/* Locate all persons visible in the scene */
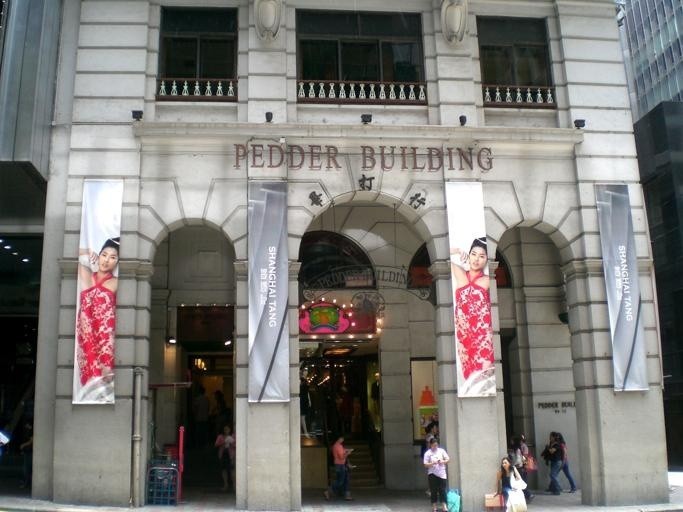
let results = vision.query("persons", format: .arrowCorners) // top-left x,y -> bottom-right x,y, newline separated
518,433 -> 530,456
299,374 -> 312,435
422,422 -> 439,501
507,434 -> 534,504
423,437 -> 449,512
333,393 -> 343,416
449,235 -> 496,398
74,236 -> 119,405
495,457 -> 528,512
544,431 -> 577,494
322,434 -> 357,501
214,423 -> 237,494
545,431 -> 564,496
16,417 -> 33,491
370,371 -> 380,434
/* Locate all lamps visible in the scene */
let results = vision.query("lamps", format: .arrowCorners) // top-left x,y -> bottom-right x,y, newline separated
265,112 -> 274,124
132,110 -> 143,121
362,113 -> 372,125
250,0 -> 282,40
459,115 -> 466,126
436,0 -> 471,43
574,119 -> 585,130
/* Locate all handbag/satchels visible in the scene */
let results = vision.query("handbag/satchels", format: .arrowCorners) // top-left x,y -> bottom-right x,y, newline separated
447,489 -> 460,512
510,471 -> 528,490
